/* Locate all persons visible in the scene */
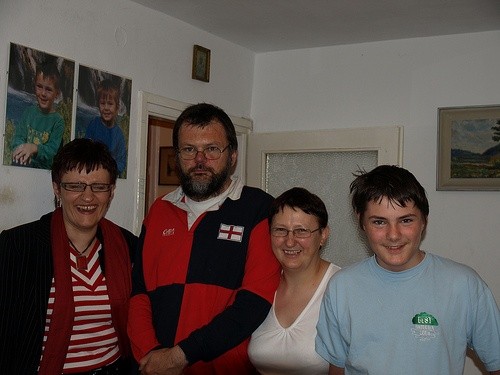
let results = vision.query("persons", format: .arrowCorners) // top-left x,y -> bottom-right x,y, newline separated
315,165 -> 500,375
247,187 -> 343,375
12,62 -> 64,170
127,103 -> 282,375
85,78 -> 126,174
0,138 -> 139,374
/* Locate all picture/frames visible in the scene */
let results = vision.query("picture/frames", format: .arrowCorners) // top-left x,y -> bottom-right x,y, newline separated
436,105 -> 500,192
158,146 -> 180,186
191,44 -> 211,82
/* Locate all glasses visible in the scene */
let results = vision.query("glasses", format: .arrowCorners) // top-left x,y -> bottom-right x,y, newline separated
60,183 -> 112,192
270,228 -> 320,238
177,144 -> 229,160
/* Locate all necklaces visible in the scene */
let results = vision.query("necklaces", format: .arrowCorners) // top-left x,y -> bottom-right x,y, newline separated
69,234 -> 96,270
